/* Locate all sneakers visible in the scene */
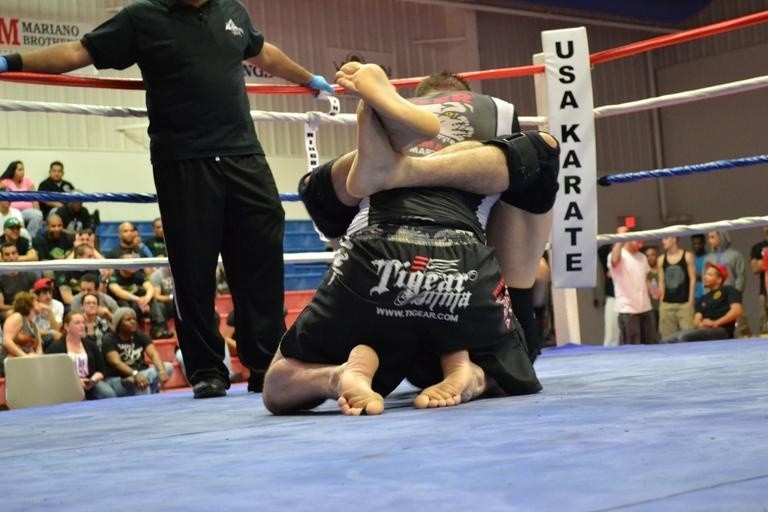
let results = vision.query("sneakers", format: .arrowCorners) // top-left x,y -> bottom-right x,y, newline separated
151,327 -> 174,339
120,376 -> 135,389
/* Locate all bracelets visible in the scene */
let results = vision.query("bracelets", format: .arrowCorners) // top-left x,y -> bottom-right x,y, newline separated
3,52 -> 22,73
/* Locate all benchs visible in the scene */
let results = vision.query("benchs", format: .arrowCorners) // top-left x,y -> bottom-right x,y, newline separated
1,217 -> 335,406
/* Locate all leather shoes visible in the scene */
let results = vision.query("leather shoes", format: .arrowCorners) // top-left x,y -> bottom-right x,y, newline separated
193,378 -> 227,398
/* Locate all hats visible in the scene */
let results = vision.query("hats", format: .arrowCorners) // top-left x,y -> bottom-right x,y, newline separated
32,278 -> 55,292
4,217 -> 22,228
705,260 -> 728,280
112,307 -> 136,330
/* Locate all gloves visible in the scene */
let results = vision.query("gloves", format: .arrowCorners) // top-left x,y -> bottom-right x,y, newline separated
307,74 -> 335,97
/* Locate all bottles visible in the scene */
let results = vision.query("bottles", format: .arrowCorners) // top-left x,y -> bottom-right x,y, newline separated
650,278 -> 658,300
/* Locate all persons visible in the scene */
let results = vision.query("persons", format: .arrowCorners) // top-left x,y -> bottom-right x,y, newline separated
1,161 -> 74,237
297,63 -> 562,386
605,220 -> 768,343
1,186 -> 287,400
2,0 -> 335,400
